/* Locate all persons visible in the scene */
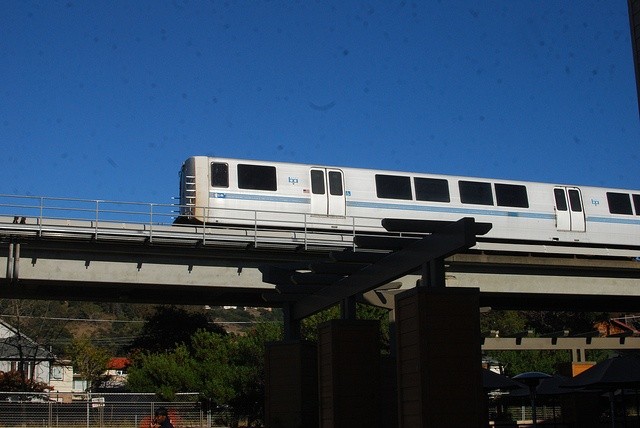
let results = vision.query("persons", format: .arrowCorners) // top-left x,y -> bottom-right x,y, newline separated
155,408 -> 174,428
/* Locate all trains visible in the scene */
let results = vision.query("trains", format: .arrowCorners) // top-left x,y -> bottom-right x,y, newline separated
170,155 -> 640,264
